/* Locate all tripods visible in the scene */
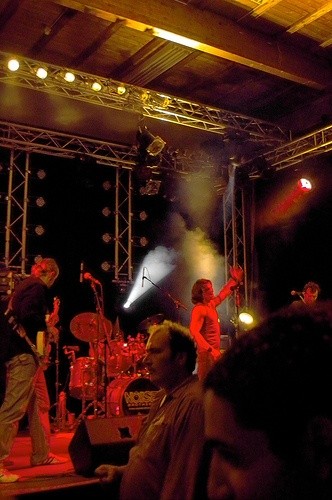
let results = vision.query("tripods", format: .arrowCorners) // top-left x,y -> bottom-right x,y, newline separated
47,282 -> 114,433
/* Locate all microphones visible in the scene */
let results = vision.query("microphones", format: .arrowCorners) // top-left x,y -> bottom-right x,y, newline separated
84,272 -> 101,286
291,291 -> 307,296
142,268 -> 144,287
79,262 -> 83,282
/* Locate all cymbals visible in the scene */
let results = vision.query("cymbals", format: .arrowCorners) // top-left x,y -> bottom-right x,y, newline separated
138,314 -> 163,330
69,312 -> 113,343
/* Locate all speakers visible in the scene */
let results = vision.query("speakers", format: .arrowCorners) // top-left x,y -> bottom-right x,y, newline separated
69,415 -> 147,479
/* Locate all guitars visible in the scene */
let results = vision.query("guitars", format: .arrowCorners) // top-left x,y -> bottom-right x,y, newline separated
36,297 -> 60,370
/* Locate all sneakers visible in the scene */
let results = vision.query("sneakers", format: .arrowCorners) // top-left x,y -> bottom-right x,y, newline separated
0,468 -> 19,483
31,452 -> 68,465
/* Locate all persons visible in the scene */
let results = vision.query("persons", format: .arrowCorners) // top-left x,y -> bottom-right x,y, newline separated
204,307 -> 332,500
95,321 -> 205,500
0,258 -> 60,483
189,265 -> 243,381
289,282 -> 320,307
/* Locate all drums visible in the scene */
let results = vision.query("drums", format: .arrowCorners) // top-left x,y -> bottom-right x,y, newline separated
88,338 -> 148,377
103,375 -> 160,416
69,357 -> 105,399
